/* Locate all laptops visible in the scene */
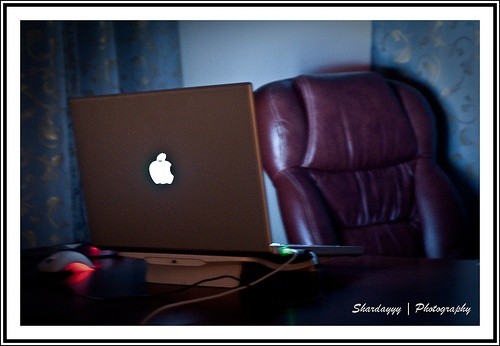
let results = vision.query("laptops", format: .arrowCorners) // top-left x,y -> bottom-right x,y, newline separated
65,78 -> 364,271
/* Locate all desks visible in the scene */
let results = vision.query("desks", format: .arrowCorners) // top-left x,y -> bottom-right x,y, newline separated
20,243 -> 479,325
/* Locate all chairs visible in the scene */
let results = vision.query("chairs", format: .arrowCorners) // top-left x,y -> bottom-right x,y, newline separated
252,72 -> 461,260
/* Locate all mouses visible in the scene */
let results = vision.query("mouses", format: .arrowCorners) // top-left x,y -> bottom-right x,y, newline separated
34,247 -> 98,278
